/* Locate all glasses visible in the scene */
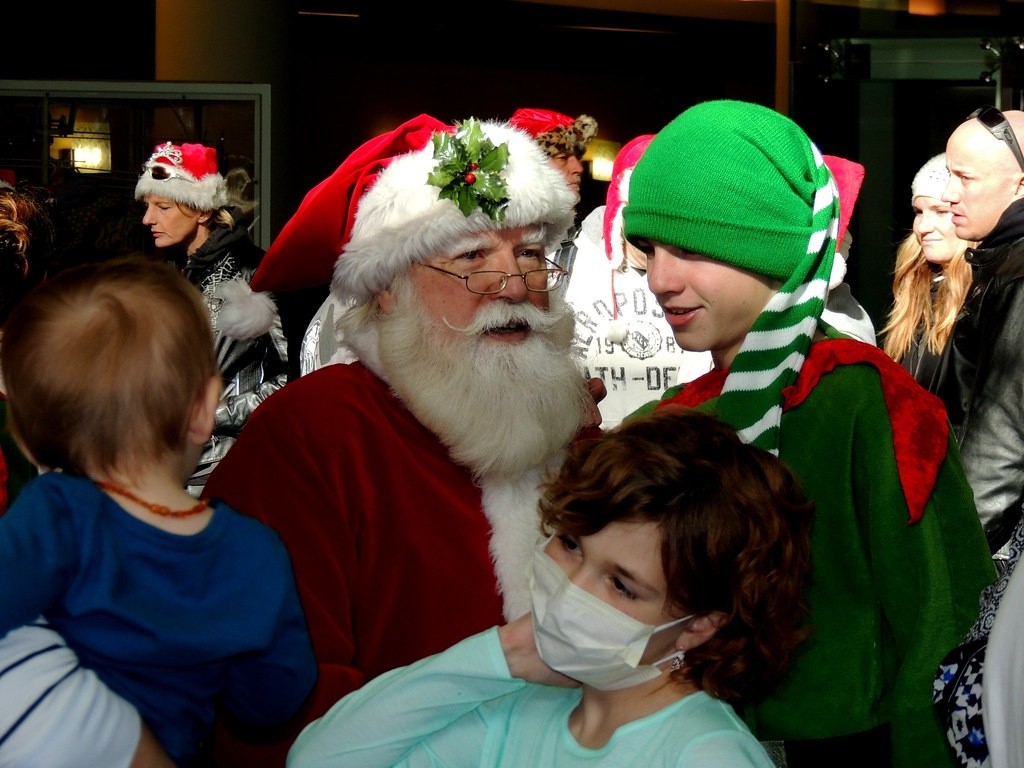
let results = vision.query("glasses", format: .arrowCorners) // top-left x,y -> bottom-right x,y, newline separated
139,163 -> 195,182
969,105 -> 1023,173
415,257 -> 571,294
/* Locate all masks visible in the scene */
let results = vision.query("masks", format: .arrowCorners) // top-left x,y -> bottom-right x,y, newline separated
523,530 -> 693,691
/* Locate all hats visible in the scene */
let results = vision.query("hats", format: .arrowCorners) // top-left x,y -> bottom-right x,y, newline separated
910,151 -> 950,201
822,154 -> 866,290
511,107 -> 596,155
217,111 -> 578,342
605,133 -> 656,268
134,143 -> 228,209
622,97 -> 843,462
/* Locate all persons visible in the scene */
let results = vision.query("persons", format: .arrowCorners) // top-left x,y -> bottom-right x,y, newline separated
287,411 -> 819,768
134,142 -> 302,498
203,113 -> 592,768
936,105 -> 1024,560
884,152 -> 982,390
0,255 -> 319,768
507,106 -> 599,201
585,99 -> 1000,768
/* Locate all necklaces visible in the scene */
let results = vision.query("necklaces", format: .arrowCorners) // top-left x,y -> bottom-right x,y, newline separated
97,481 -> 209,518
